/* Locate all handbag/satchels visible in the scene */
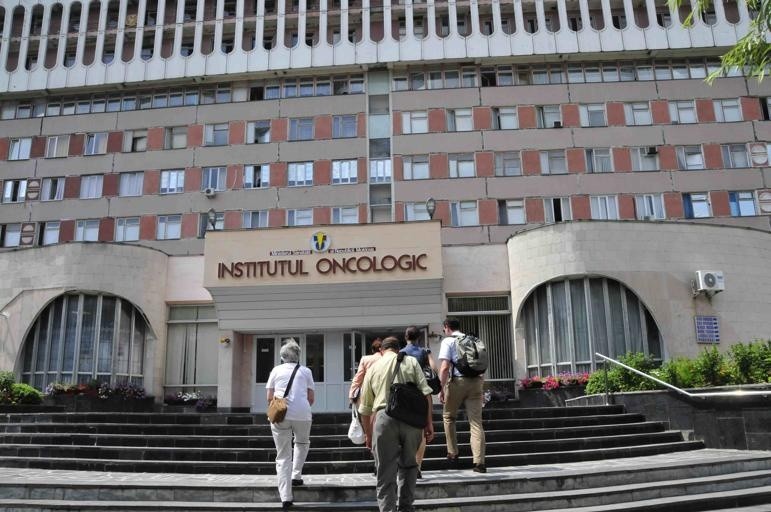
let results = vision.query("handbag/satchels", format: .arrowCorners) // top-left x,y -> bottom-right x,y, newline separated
348,403 -> 366,445
385,382 -> 428,429
422,366 -> 442,395
348,387 -> 360,409
267,397 -> 287,424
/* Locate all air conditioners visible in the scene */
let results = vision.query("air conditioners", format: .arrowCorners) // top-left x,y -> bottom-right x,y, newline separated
693,269 -> 725,293
648,146 -> 658,153
204,187 -> 216,197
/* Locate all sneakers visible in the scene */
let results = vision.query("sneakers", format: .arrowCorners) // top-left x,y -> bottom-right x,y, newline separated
283,501 -> 293,505
417,469 -> 422,478
447,454 -> 458,460
473,465 -> 487,473
292,479 -> 304,486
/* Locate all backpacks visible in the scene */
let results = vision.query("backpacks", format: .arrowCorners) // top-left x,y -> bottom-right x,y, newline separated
446,333 -> 488,375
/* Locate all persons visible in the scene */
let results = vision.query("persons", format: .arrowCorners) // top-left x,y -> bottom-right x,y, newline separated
358,336 -> 434,512
399,325 -> 437,478
265,337 -> 315,507
439,316 -> 488,473
348,339 -> 384,475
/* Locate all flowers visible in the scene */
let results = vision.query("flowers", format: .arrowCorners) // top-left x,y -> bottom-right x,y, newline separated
518,370 -> 589,391
46,378 -> 214,411
